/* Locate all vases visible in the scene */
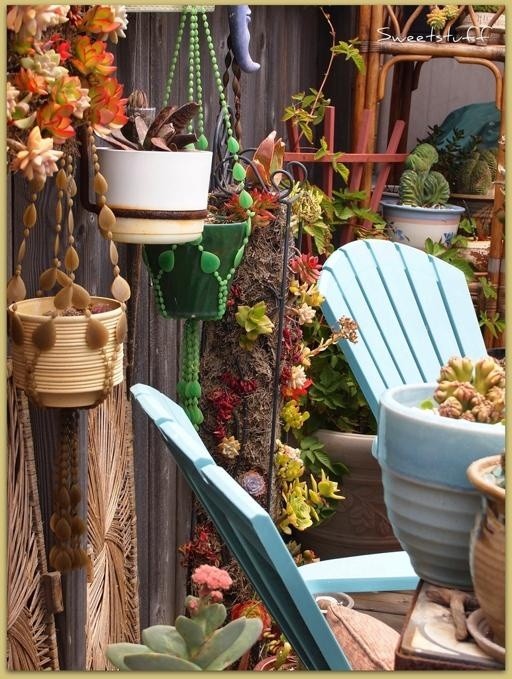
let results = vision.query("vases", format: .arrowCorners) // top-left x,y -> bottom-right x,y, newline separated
7,294 -> 126,410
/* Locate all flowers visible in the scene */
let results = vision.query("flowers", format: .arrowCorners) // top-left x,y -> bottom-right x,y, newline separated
6,4 -> 128,181
